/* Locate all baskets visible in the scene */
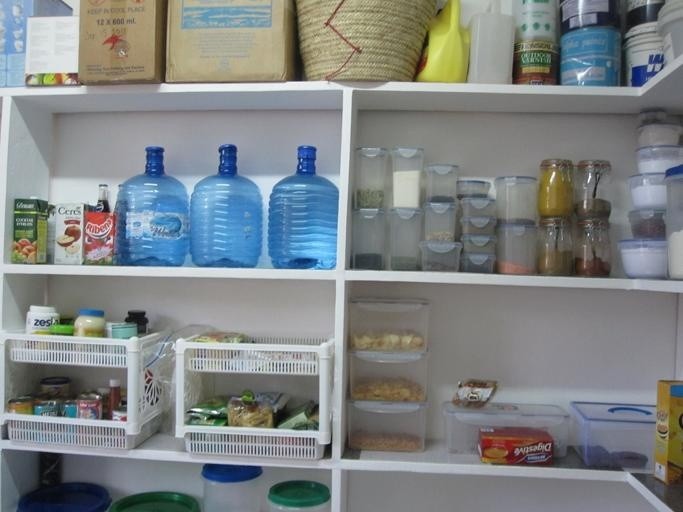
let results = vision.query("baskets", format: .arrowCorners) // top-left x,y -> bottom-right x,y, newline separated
297,0 -> 438,82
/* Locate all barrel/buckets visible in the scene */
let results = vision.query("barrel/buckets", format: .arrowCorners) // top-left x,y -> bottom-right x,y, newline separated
623,24 -> 662,86
468,0 -> 516,83
202,463 -> 266,512
417,2 -> 468,82
657,0 -> 683,65
16,483 -> 108,512
266,480 -> 331,511
109,491 -> 200,512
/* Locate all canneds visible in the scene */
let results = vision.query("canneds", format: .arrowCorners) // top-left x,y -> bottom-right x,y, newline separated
112,405 -> 127,421
538,158 -> 613,277
8,376 -> 103,419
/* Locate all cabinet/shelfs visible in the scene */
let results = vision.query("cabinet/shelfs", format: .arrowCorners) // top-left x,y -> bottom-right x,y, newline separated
0,54 -> 683,512
77,0 -> 297,86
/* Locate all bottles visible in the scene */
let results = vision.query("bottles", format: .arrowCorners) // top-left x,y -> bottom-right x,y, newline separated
107,376 -> 122,420
95,183 -> 111,214
572,157 -> 613,219
571,220 -> 614,278
535,157 -> 576,218
26,304 -> 149,341
534,214 -> 575,277
